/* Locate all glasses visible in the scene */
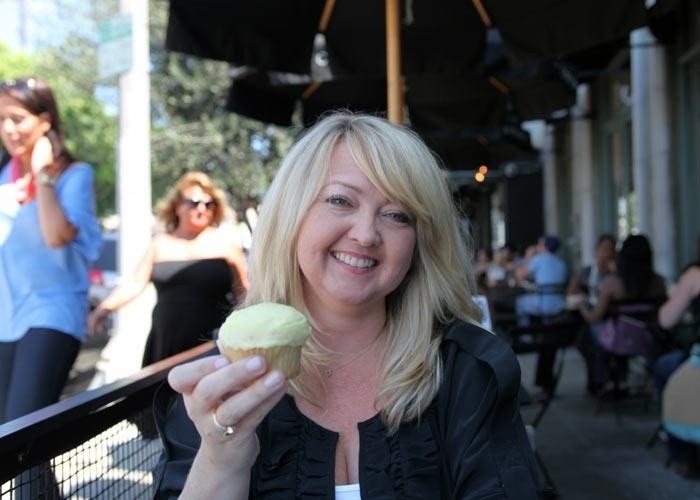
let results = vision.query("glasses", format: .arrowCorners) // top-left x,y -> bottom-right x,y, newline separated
182,199 -> 214,211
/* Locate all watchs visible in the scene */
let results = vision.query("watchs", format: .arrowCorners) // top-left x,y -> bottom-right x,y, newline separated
33,174 -> 54,186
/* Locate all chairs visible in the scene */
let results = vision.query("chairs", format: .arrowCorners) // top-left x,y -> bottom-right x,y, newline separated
484,283 -> 699,477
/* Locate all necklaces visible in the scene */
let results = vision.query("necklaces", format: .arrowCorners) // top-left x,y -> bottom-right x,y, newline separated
319,342 -> 372,376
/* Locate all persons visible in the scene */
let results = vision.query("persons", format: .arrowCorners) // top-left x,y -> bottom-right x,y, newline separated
153,109 -> 560,500
474,235 -> 699,477
87,171 -> 249,440
0,78 -> 103,500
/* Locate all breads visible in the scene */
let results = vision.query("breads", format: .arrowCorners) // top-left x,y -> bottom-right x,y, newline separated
216,300 -> 311,380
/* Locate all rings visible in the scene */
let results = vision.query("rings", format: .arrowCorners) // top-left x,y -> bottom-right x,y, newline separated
213,409 -> 237,437
